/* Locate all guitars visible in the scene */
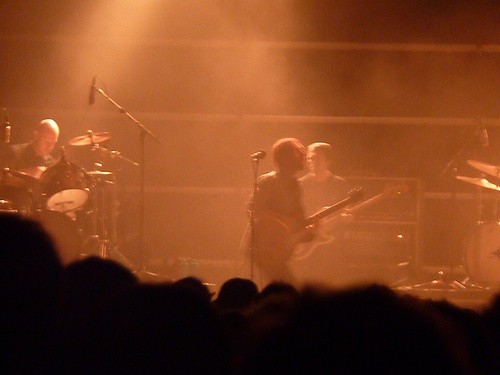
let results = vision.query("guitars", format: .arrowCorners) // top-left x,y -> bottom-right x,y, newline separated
253,183 -> 407,261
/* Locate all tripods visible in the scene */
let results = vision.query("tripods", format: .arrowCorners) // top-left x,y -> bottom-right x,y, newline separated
80,176 -> 130,268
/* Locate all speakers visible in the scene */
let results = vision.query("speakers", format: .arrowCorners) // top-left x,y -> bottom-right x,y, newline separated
332,219 -> 418,290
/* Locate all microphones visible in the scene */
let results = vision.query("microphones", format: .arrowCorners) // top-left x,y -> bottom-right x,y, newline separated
480,123 -> 489,146
60,146 -> 65,158
89,77 -> 97,105
4,113 -> 11,143
249,150 -> 266,160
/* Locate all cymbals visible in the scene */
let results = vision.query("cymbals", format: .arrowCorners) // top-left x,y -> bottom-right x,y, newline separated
68,132 -> 112,146
467,159 -> 500,178
78,169 -> 123,178
455,175 -> 500,191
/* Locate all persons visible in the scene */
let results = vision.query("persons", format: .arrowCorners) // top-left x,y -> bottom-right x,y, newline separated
240,138 -> 322,282
298,142 -> 353,284
0,211 -> 500,375
5,118 -> 60,170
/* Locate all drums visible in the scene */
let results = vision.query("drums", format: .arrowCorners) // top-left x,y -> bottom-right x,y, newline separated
46,188 -> 96,239
464,219 -> 500,287
13,209 -> 82,265
